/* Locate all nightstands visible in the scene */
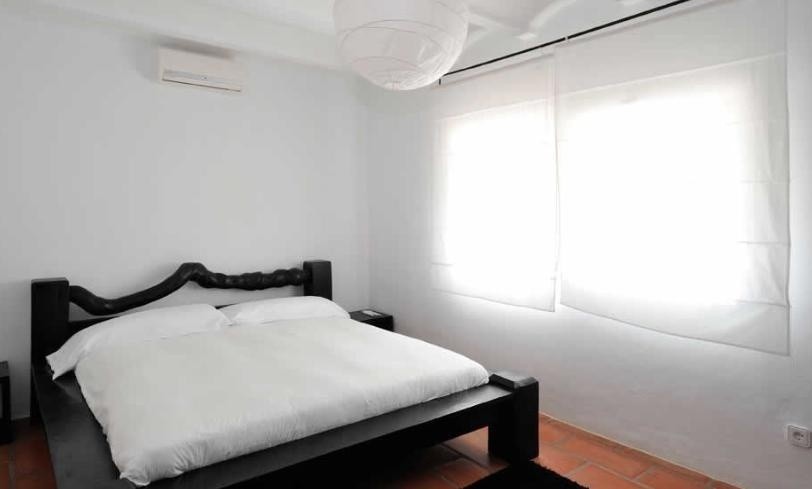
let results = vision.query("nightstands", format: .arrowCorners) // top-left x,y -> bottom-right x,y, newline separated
0,360 -> 14,445
348,309 -> 393,331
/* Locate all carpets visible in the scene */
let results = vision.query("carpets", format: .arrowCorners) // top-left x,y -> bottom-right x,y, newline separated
462,459 -> 589,489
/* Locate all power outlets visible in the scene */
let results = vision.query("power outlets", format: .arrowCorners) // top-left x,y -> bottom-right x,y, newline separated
785,424 -> 812,448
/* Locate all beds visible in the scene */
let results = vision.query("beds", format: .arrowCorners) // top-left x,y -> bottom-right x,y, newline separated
29,259 -> 539,489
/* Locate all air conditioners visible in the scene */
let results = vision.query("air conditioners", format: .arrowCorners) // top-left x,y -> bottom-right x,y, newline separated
156,48 -> 243,95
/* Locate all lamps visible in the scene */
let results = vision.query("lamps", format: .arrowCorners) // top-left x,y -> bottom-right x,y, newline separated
330,0 -> 468,90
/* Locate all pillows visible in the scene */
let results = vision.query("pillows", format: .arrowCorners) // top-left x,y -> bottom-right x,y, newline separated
45,303 -> 232,381
217,296 -> 351,327
